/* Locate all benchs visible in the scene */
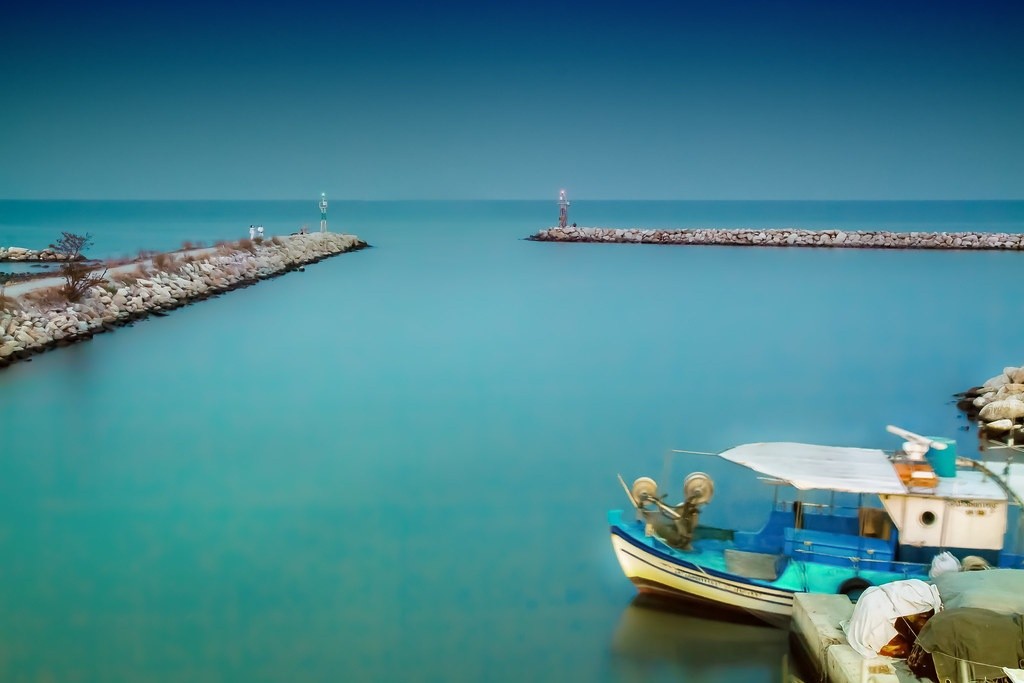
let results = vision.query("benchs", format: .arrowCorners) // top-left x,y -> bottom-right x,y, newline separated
782,527 -> 891,569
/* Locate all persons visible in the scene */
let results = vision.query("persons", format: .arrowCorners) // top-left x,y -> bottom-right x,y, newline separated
257,224 -> 264,237
248,224 -> 256,240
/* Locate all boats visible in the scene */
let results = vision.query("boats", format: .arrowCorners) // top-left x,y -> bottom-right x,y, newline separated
607,424 -> 1023,629
